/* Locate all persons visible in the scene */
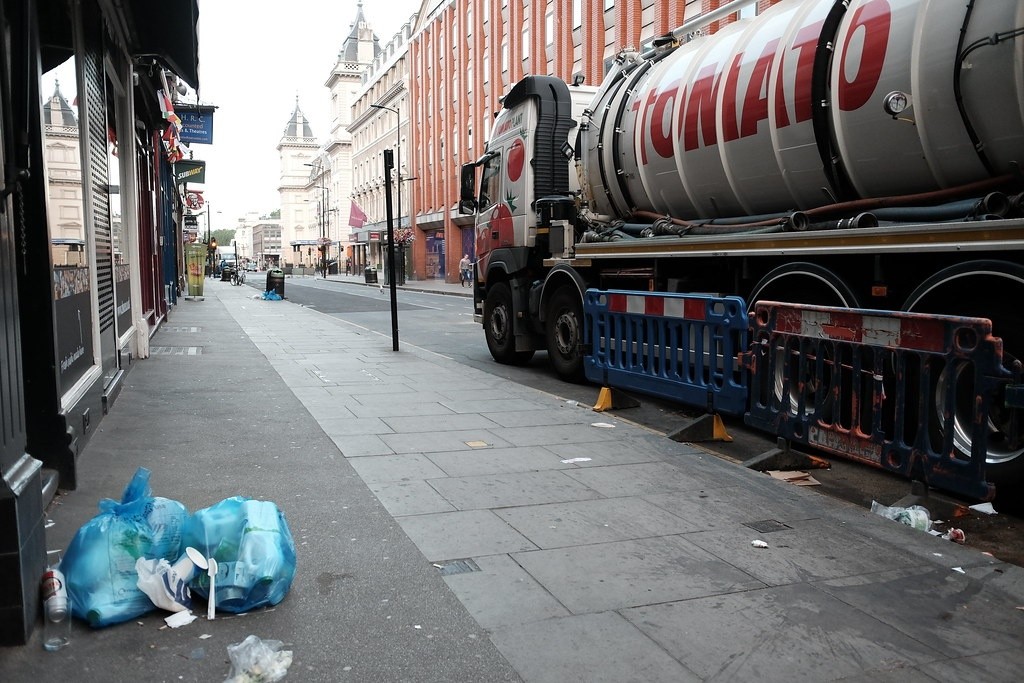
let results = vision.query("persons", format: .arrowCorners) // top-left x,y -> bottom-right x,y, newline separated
215,259 -> 226,277
459,254 -> 473,288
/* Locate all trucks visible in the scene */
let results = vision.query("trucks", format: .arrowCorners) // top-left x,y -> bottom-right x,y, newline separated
218,252 -> 240,271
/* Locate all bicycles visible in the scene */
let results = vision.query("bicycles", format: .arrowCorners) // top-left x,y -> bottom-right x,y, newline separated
231,270 -> 246,287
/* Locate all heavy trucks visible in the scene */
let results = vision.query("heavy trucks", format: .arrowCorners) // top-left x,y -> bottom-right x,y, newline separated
459,0 -> 1024,512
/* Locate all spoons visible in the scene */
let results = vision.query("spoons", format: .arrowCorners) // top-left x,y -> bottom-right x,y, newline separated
207,558 -> 218,621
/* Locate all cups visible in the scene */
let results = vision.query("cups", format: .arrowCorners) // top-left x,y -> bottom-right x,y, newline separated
171,547 -> 207,584
43,596 -> 73,651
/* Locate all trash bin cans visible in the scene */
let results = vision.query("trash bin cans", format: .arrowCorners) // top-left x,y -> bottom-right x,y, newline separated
219,266 -> 231,281
364,267 -> 378,283
266,268 -> 284,300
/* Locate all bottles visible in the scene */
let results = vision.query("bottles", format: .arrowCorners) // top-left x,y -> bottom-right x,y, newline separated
42,570 -> 68,621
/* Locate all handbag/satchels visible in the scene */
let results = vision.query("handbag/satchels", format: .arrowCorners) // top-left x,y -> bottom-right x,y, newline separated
179,494 -> 300,613
59,466 -> 188,629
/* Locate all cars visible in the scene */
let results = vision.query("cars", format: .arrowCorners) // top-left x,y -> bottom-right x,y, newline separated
246,262 -> 257,272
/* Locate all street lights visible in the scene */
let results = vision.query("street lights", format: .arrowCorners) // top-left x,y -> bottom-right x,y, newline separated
370,104 -> 402,229
301,163 -> 335,279
